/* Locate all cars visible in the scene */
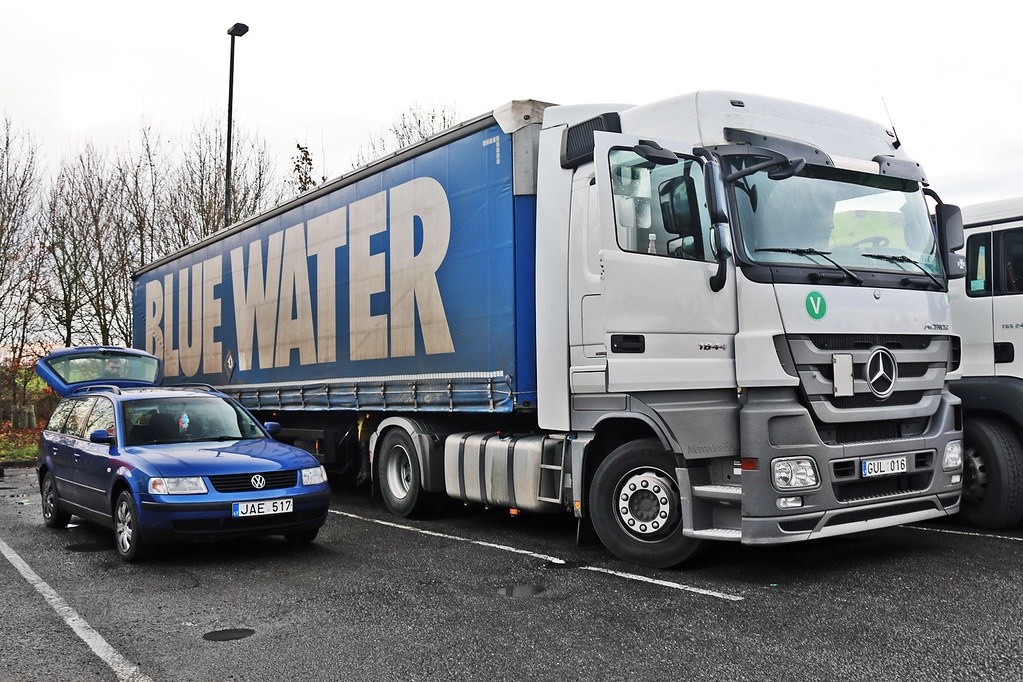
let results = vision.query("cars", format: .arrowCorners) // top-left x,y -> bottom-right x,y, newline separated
35,345 -> 331,563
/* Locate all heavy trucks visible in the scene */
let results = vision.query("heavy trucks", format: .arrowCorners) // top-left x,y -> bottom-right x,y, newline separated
129,90 -> 1023,567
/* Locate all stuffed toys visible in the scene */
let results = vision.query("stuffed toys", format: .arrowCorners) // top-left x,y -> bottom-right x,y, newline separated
179,414 -> 189,429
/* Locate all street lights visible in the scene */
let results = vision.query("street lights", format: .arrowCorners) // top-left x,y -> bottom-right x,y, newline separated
224,23 -> 249,228
